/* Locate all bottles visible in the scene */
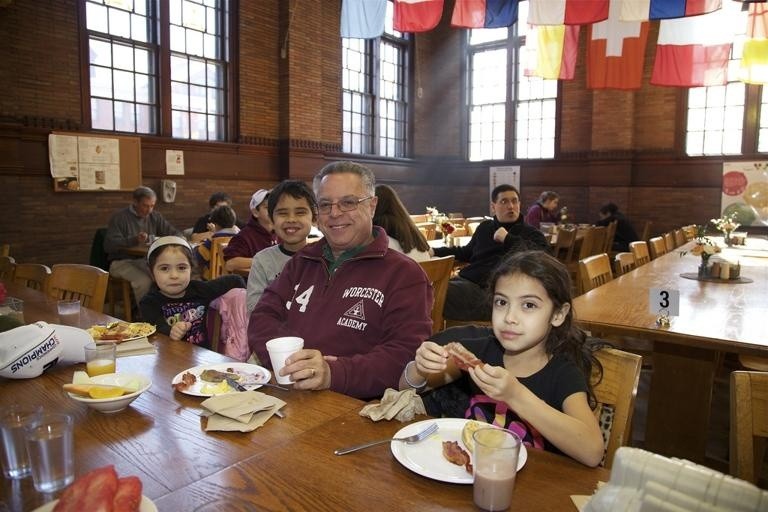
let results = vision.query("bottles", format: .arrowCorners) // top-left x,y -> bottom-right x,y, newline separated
710,262 -> 730,281
447,233 -> 461,247
732,235 -> 741,245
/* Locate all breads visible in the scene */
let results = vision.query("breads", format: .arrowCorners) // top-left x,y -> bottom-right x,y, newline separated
460,419 -> 501,457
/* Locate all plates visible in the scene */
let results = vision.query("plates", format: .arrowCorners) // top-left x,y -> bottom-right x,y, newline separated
389,417 -> 528,487
31,493 -> 159,512
94,321 -> 157,344
170,362 -> 272,399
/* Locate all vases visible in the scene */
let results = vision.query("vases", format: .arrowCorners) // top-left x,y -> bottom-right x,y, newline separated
698,262 -> 710,279
723,234 -> 734,247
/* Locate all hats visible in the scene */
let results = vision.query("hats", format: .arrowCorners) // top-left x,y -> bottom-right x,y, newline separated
249,189 -> 274,210
0,321 -> 96,380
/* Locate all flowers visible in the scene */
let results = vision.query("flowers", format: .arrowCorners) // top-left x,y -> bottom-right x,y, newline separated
677,235 -> 722,261
708,210 -> 743,234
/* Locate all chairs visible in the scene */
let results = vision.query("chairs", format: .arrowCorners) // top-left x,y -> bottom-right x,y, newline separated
584,342 -> 643,470
569,222 -> 702,296
107,209 -> 624,359
725,367 -> 767,486
1,256 -> 110,314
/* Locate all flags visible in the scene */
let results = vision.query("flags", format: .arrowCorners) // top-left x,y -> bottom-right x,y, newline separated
335,1 -> 768,89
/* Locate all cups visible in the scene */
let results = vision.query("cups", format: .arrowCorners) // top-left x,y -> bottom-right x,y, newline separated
56,299 -> 81,328
263,335 -> 305,387
84,340 -> 117,377
469,427 -> 521,512
0,403 -> 76,494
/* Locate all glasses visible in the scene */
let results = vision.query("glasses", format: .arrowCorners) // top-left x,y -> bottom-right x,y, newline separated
316,195 -> 373,215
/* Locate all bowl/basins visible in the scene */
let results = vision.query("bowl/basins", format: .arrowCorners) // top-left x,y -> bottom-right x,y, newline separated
66,371 -> 154,414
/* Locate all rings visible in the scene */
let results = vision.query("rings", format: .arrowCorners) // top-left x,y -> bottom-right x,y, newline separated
310,369 -> 316,378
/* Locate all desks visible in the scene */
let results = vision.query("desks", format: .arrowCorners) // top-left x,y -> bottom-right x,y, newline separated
1,277 -> 611,512
569,233 -> 768,469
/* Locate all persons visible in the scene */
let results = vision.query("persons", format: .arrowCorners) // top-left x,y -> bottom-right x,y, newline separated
248,182 -> 319,316
590,202 -> 638,259
223,188 -> 280,271
426,184 -> 553,323
106,186 -> 186,322
247,162 -> 434,399
139,237 -> 247,352
396,249 -> 605,468
190,192 -> 247,282
524,190 -> 570,231
372,183 -> 430,262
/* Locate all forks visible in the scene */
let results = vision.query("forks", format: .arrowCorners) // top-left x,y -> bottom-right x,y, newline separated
331,423 -> 440,457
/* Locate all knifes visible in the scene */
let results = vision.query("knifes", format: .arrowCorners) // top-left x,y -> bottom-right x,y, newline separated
224,375 -> 290,421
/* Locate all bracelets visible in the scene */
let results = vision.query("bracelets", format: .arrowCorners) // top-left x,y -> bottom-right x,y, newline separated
402,360 -> 429,389
